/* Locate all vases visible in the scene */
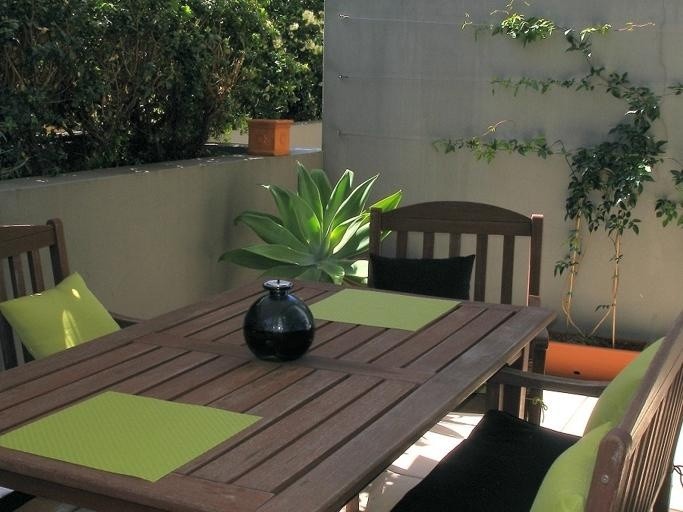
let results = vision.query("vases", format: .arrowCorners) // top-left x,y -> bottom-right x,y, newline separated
242,279 -> 314,360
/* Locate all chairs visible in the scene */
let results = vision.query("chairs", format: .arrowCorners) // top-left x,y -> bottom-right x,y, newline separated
0,217 -> 141,369
393,308 -> 682,511
344,203 -> 548,510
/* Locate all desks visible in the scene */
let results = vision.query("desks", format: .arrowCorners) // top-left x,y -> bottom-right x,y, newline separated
0,283 -> 555,511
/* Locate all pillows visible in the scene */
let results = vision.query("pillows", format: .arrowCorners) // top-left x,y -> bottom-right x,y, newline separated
364,252 -> 472,300
0,273 -> 121,363
581,336 -> 665,438
528,421 -> 612,512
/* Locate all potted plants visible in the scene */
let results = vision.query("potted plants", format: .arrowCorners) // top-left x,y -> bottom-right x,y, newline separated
420,0 -> 682,380
231,46 -> 311,157
